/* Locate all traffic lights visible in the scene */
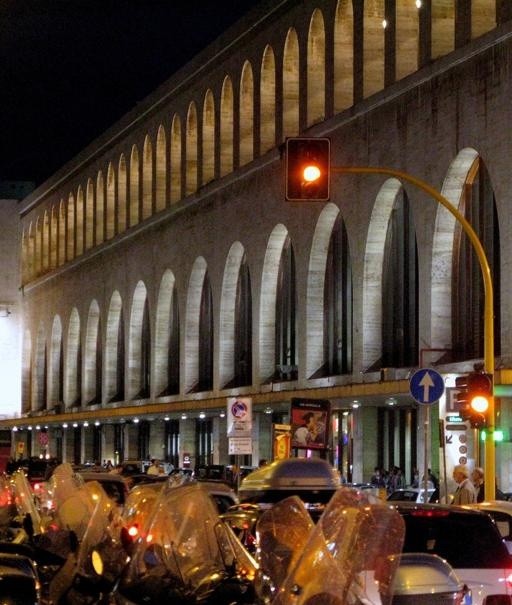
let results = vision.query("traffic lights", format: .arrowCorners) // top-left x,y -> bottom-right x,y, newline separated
467,373 -> 496,430
454,375 -> 472,424
285,135 -> 332,203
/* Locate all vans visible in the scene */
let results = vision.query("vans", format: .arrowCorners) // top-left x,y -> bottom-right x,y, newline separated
391,496 -> 512,605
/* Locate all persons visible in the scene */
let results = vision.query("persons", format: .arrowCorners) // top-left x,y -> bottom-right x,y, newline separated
294,412 -> 325,446
371,465 -> 439,503
147,460 -> 164,476
471,468 -> 484,503
84,459 -> 114,473
452,465 -> 477,505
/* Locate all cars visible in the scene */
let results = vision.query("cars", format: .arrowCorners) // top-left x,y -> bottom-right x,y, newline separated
462,497 -> 512,556
